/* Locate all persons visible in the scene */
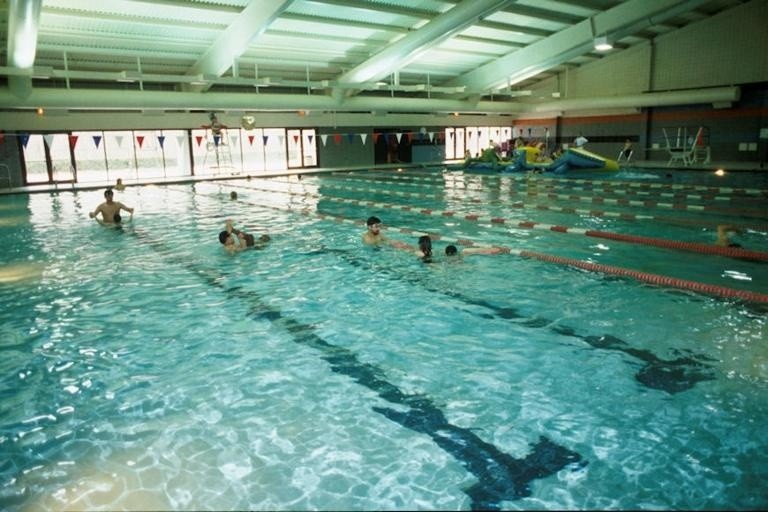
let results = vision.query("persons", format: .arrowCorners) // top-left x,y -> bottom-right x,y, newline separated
464,131 -> 590,163
219,219 -> 271,248
86,189 -> 135,229
624,138 -> 632,158
714,223 -> 745,250
443,245 -> 504,256
89,210 -> 134,226
362,216 -> 387,244
415,235 -> 432,256
224,234 -> 247,253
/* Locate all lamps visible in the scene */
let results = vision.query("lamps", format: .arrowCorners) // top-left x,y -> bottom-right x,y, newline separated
592,35 -> 616,54
